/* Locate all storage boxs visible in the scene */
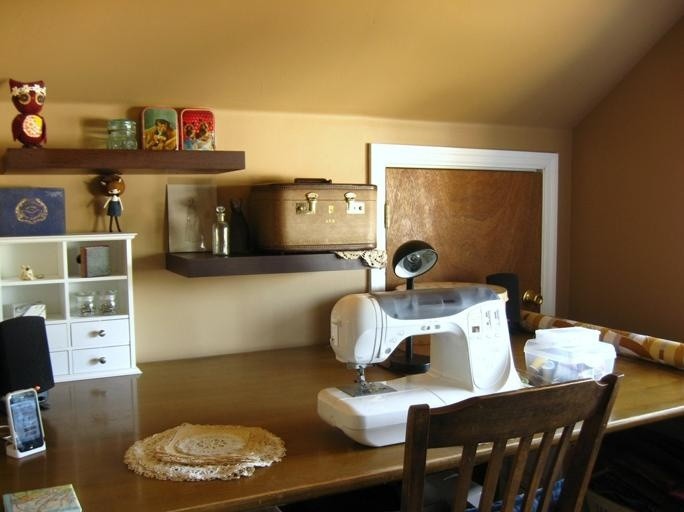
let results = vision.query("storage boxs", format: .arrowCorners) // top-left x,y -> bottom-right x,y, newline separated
248,179 -> 376,254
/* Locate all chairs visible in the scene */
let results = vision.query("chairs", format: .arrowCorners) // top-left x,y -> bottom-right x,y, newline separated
398,371 -> 624,512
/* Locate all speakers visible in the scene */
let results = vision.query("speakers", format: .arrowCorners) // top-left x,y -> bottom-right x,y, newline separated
487,273 -> 519,330
0,316 -> 55,409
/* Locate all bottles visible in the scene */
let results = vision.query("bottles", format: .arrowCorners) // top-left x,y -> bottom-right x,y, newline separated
229,197 -> 243,251
94,289 -> 119,314
76,295 -> 95,316
107,120 -> 137,150
211,206 -> 231,256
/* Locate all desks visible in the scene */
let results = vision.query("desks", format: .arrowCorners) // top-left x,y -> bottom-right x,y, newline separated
2,327 -> 683,510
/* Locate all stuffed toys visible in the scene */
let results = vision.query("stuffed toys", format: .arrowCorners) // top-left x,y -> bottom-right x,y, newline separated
9,78 -> 49,149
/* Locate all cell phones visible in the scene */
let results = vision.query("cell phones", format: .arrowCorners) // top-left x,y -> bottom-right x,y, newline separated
5,388 -> 44,452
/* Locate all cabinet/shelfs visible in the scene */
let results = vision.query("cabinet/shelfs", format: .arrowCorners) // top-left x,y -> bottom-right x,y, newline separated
368,142 -> 557,345
1,147 -> 373,276
0,233 -> 145,384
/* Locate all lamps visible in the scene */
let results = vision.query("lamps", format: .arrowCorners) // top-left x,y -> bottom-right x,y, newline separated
391,239 -> 440,375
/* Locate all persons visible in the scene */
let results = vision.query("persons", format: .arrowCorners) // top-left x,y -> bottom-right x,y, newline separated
99,173 -> 125,235
